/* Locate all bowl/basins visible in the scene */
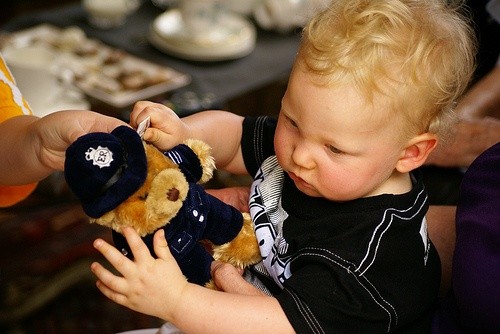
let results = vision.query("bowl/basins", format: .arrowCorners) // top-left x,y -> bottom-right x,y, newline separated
81,0 -> 141,30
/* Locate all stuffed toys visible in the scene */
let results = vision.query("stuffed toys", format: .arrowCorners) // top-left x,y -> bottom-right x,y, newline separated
64,125 -> 263,291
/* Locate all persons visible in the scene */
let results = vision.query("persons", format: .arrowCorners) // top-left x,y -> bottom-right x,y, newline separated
90,0 -> 500,334
0,54 -> 133,209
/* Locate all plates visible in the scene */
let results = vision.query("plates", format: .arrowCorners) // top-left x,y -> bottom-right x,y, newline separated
148,6 -> 256,61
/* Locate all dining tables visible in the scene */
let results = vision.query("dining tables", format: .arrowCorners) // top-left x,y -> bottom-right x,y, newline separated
0,0 -> 304,123
